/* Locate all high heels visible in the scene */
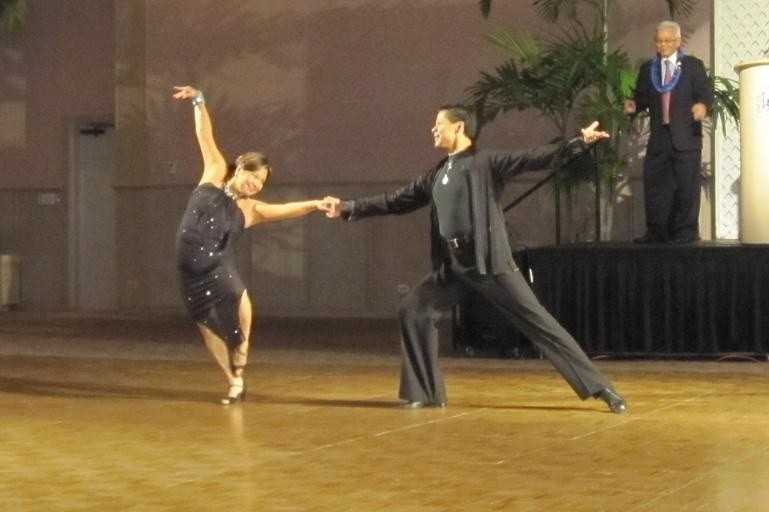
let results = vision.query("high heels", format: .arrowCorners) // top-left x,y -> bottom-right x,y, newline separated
217,375 -> 249,406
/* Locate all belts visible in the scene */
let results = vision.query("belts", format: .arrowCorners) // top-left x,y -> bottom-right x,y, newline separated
436,236 -> 477,254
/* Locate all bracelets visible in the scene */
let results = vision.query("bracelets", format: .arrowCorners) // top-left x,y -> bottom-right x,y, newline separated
193,88 -> 206,105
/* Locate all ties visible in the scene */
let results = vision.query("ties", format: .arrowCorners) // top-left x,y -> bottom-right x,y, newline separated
660,60 -> 674,127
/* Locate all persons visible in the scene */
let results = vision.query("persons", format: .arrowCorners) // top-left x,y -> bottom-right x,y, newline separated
169,80 -> 333,407
326,104 -> 632,415
624,20 -> 716,245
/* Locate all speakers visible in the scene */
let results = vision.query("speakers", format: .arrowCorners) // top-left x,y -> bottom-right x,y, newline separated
454,249 -> 528,349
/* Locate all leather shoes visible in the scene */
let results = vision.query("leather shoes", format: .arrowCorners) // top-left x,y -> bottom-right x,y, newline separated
231,342 -> 249,376
662,231 -> 701,245
397,396 -> 449,410
599,383 -> 629,413
632,230 -> 666,244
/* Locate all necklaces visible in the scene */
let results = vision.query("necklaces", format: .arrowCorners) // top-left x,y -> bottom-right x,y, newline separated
225,179 -> 245,202
